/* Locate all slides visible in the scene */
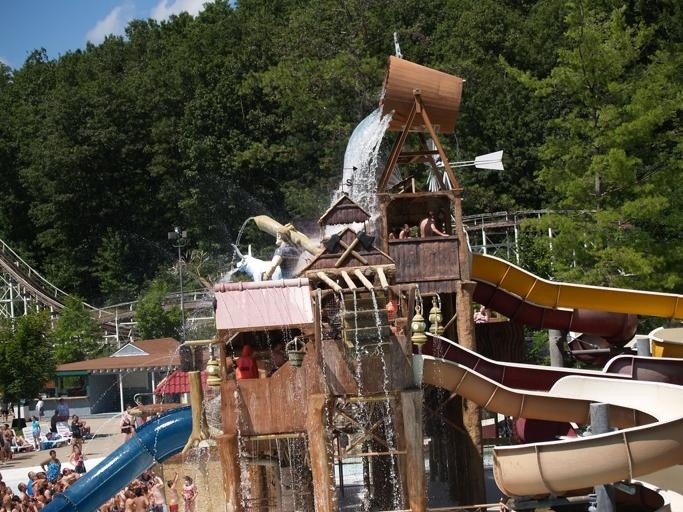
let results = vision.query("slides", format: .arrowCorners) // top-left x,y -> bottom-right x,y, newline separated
468,247 -> 683,365
421,333 -> 683,512
38,405 -> 192,512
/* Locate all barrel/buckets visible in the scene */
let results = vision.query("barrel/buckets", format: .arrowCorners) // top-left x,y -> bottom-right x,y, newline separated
652,327 -> 682,357
286,337 -> 308,367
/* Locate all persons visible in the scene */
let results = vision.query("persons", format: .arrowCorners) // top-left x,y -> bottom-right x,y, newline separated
473,305 -> 490,324
0,396 -> 198,512
263,222 -> 306,280
235,345 -> 259,379
388,210 -> 451,239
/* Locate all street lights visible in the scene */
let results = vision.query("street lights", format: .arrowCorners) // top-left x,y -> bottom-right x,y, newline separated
166,226 -> 191,341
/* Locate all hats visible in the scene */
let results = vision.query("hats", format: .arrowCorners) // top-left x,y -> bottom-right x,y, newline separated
243,345 -> 251,355
